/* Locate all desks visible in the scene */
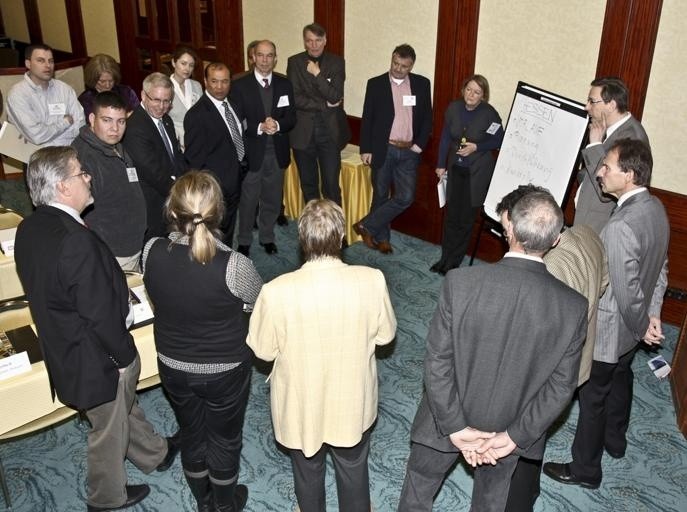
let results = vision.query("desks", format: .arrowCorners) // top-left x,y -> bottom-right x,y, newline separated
0,204 -> 162,441
277,143 -> 389,247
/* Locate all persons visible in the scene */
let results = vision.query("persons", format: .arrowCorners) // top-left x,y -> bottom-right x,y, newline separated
396,189 -> 591,512
11,146 -> 180,512
572,76 -> 653,238
243,197 -> 396,512
352,43 -> 431,253
543,135 -> 671,492
428,74 -> 505,277
8,43 -> 85,207
141,170 -> 262,512
286,24 -> 349,251
71,40 -> 297,275
494,184 -> 612,511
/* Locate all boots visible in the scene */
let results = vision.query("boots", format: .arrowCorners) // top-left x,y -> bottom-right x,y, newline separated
183,468 -> 248,512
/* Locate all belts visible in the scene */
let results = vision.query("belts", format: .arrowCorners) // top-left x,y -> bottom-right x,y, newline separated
389,140 -> 414,148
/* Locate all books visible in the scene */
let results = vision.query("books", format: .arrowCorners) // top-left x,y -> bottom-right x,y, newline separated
436,170 -> 448,209
0,328 -> 15,361
127,283 -> 155,325
5,323 -> 45,365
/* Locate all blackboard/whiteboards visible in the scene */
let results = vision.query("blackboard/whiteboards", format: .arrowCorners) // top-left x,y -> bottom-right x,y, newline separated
479,81 -> 590,235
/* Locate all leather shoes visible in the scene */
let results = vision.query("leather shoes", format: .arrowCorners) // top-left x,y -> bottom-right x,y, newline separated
543,462 -> 600,489
156,433 -> 179,472
259,241 -> 277,254
277,214 -> 288,226
429,257 -> 458,276
353,222 -> 378,250
237,245 -> 250,258
87,483 -> 150,511
376,240 -> 392,255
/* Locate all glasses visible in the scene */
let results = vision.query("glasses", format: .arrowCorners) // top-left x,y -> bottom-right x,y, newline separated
587,98 -> 603,105
67,171 -> 87,178
143,87 -> 172,105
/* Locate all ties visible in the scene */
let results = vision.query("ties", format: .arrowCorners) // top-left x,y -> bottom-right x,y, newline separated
158,120 -> 175,165
263,79 -> 269,87
222,101 -> 245,163
